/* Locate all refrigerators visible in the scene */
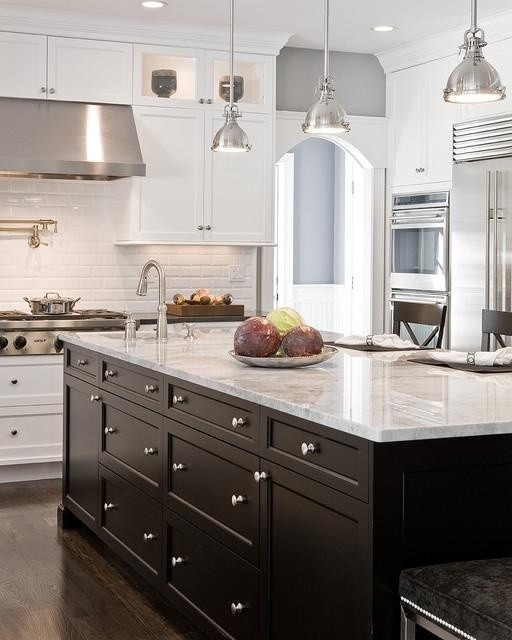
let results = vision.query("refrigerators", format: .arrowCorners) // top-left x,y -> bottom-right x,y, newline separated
449,112 -> 512,352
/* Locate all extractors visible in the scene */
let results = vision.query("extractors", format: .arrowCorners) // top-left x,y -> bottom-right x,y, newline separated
0,96 -> 148,183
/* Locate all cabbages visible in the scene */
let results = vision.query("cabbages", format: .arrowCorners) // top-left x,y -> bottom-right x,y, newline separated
265,307 -> 303,341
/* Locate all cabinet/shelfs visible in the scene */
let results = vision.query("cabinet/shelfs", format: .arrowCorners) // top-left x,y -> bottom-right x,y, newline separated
0,358 -> 61,476
101,351 -> 164,595
267,410 -> 511,640
165,376 -> 267,636
115,112 -> 278,248
118,44 -> 278,113
55,336 -> 101,541
0,21 -> 131,110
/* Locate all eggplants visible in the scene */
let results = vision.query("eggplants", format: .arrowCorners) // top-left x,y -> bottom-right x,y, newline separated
222,293 -> 233,305
174,293 -> 185,305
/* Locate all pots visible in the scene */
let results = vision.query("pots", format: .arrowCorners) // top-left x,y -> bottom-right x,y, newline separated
22,292 -> 82,315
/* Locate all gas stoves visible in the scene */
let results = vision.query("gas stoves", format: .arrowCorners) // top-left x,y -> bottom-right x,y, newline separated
0,309 -> 127,356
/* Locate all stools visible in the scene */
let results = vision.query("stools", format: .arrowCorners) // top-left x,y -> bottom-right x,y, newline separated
398,558 -> 511,638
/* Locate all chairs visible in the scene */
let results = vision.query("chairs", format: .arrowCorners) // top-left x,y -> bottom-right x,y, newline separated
386,302 -> 446,349
478,308 -> 512,356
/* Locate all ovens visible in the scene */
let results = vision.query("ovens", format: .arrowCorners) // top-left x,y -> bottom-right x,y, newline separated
390,191 -> 450,350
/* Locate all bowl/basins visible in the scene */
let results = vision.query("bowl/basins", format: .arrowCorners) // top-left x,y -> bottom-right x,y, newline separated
217,76 -> 246,103
151,69 -> 176,98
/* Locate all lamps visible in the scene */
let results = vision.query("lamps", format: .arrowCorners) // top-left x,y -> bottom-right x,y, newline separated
300,1 -> 352,135
443,2 -> 507,102
211,0 -> 252,155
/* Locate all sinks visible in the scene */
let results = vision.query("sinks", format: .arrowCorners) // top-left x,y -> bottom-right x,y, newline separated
96,331 -> 185,340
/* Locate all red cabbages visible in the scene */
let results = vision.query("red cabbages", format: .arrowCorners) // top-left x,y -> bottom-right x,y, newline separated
234,317 -> 281,356
281,326 -> 324,356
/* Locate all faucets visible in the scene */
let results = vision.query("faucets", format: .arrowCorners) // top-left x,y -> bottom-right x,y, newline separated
135,259 -> 168,339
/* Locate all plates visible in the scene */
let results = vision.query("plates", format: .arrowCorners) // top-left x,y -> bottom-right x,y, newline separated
228,345 -> 337,370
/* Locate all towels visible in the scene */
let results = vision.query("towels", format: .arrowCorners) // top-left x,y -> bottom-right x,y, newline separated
334,332 -> 422,353
426,349 -> 511,369
